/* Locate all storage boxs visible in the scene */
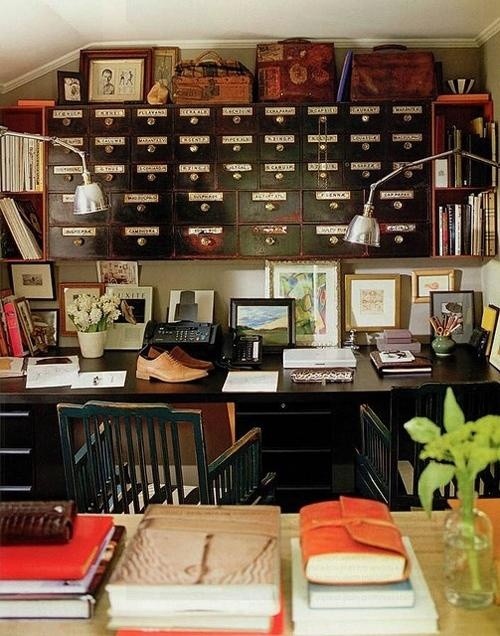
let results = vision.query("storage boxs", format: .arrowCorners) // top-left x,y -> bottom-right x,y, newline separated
172,41 -> 437,105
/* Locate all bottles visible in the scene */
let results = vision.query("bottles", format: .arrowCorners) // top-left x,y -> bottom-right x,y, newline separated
433,334 -> 457,357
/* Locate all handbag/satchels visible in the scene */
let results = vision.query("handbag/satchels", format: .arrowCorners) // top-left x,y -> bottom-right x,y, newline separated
174,52 -> 253,76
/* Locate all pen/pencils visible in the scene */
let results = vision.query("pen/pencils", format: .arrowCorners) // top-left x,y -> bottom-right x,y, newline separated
429,311 -> 464,336
94,376 -> 98,383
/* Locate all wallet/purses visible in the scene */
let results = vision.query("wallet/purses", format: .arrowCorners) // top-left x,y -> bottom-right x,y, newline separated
0,502 -> 76,543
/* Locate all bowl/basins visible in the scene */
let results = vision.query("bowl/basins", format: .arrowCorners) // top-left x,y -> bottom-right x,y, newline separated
444,77 -> 477,94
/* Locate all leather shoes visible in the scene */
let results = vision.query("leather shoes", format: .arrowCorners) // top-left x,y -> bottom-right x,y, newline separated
136,351 -> 208,382
148,346 -> 215,371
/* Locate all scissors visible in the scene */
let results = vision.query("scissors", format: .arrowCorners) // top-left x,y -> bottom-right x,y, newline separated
436,327 -> 450,337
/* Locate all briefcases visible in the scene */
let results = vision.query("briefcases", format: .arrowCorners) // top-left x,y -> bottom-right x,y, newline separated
256,43 -> 337,103
172,76 -> 253,105
351,52 -> 438,101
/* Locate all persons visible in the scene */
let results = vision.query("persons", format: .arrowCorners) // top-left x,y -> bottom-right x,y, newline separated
100,68 -> 135,96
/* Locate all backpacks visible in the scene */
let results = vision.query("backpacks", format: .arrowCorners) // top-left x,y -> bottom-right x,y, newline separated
469,327 -> 489,353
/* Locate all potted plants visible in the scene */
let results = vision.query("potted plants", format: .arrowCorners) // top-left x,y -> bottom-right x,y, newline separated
403,387 -> 500,609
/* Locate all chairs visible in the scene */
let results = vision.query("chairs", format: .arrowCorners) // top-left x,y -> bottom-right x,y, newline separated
57,401 -> 276,513
353,382 -> 500,512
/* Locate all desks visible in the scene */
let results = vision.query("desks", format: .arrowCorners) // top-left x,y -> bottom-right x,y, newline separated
0,346 -> 500,513
0,513 -> 500,636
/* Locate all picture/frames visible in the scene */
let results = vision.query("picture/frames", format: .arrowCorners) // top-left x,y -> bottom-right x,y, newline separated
60,45 -> 179,105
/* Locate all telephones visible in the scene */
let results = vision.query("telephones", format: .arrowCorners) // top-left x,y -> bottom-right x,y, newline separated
222,331 -> 263,366
142,319 -> 222,348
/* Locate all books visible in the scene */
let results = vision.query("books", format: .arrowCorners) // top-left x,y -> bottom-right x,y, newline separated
105,501 -> 281,616
1,523 -> 127,619
0,124 -> 45,260
368,349 -> 436,379
112,579 -> 287,636
101,281 -> 154,350
0,356 -> 81,378
16,98 -> 57,106
291,533 -> 441,636
435,92 -> 489,101
481,305 -> 497,356
489,302 -> 500,356
433,109 -> 499,257
108,613 -> 274,632
0,286 -> 49,357
306,578 -> 417,610
0,508 -> 114,596
298,496 -> 411,584
